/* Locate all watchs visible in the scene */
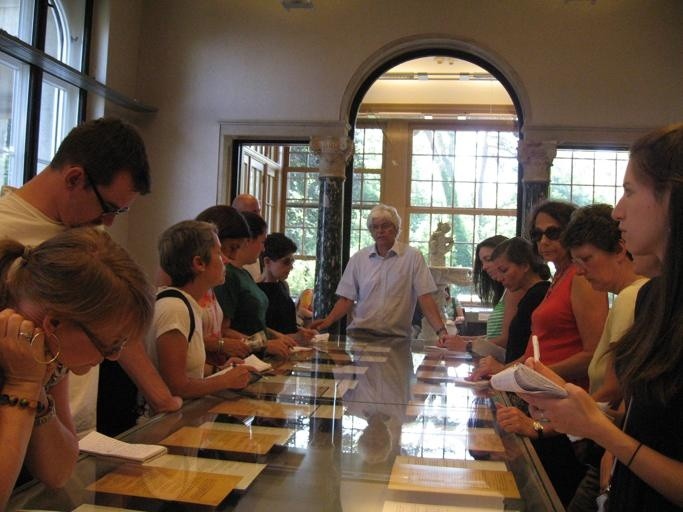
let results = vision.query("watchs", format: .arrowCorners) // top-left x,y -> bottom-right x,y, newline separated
466,341 -> 473,353
436,327 -> 447,336
533,421 -> 544,440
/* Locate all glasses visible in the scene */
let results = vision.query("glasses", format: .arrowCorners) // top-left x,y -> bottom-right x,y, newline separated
87,179 -> 128,215
282,257 -> 294,265
529,226 -> 565,241
73,319 -> 127,358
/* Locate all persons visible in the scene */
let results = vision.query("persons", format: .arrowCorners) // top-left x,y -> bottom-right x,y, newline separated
566,253 -> 664,512
466,237 -> 552,383
153,205 -> 251,377
0,226 -> 155,512
440,235 -> 526,352
444,286 -> 465,336
255,232 -> 318,347
0,117 -> 184,440
136,220 -> 258,427
213,210 -> 297,357
312,340 -> 441,465
480,201 -> 609,394
316,203 -> 448,348
231,194 -> 261,282
516,123 -> 683,512
494,204 -> 652,512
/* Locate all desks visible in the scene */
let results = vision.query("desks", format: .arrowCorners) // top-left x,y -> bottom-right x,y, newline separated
5,336 -> 568,512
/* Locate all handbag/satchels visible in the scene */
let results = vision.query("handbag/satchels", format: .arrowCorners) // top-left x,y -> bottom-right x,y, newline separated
595,493 -> 608,512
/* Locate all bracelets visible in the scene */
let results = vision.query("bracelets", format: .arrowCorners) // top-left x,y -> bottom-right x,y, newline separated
33,395 -> 56,425
626,443 -> 642,467
0,393 -> 45,413
217,336 -> 225,356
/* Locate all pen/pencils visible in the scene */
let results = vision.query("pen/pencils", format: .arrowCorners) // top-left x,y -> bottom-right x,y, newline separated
532,335 -> 540,362
230,363 -> 268,380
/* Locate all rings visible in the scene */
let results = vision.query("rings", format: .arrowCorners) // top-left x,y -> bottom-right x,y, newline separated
536,408 -> 544,419
18,332 -> 32,340
